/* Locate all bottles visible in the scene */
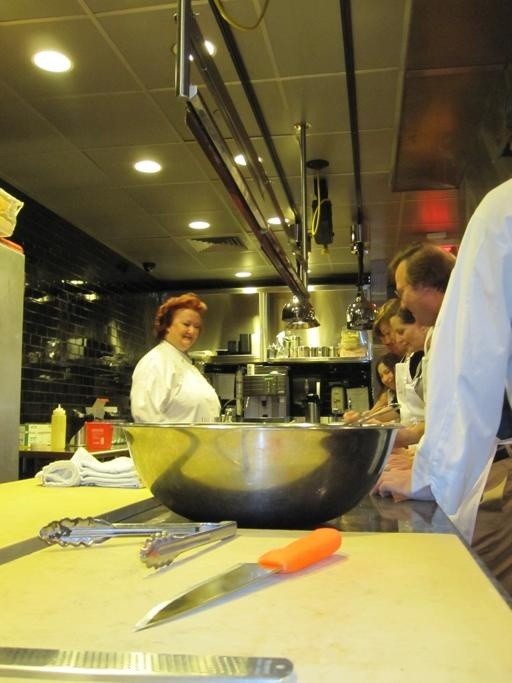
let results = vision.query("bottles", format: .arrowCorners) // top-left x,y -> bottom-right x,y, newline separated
305,393 -> 321,423
49,403 -> 66,450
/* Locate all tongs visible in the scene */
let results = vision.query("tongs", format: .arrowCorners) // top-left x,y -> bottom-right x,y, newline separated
38,515 -> 238,569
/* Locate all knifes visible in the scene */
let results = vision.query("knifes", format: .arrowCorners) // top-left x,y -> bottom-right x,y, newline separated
135,523 -> 342,628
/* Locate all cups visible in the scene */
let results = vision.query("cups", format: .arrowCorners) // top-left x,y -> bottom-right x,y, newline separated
266,334 -> 337,360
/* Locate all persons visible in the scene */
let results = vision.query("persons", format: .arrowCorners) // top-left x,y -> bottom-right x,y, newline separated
131,291 -> 221,424
370,180 -> 512,604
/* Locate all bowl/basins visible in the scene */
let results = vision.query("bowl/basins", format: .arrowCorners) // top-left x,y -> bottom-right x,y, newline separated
112,420 -> 399,528
